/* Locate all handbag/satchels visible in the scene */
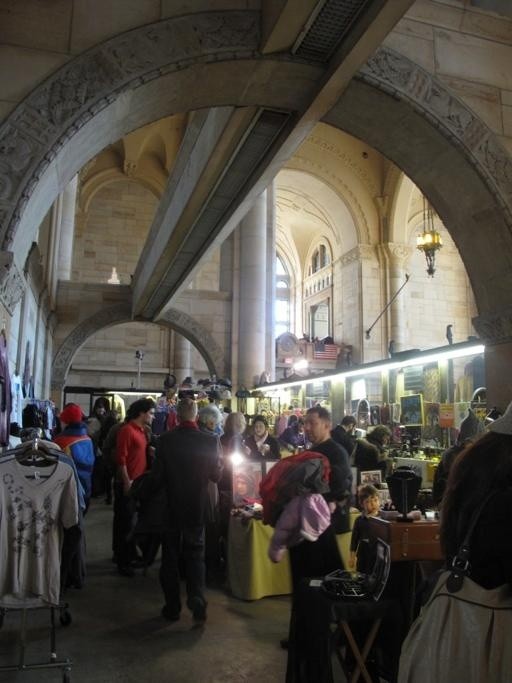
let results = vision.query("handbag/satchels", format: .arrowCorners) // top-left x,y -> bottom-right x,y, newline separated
128,471 -> 160,497
395,568 -> 512,683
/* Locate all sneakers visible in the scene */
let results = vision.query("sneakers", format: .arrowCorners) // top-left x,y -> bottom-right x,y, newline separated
104,497 -> 112,505
111,553 -> 154,578
186,595 -> 207,627
160,605 -> 181,621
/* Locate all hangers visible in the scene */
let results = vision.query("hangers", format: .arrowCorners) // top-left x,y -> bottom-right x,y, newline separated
14,433 -> 60,466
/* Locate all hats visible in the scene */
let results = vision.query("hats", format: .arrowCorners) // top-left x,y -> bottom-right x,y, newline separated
457,409 -> 485,441
487,400 -> 512,435
60,404 -> 83,425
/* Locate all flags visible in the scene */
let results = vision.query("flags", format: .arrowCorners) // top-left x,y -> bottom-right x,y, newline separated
312,341 -> 338,360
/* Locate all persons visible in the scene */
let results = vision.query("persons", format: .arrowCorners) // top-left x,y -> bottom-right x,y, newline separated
283,404 -> 394,513
278,406 -> 351,648
352,486 -> 420,574
429,403 -> 511,591
298,332 -> 334,344
50,395 -> 279,624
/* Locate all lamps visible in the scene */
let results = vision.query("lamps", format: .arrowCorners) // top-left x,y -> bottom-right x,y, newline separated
414,180 -> 443,277
